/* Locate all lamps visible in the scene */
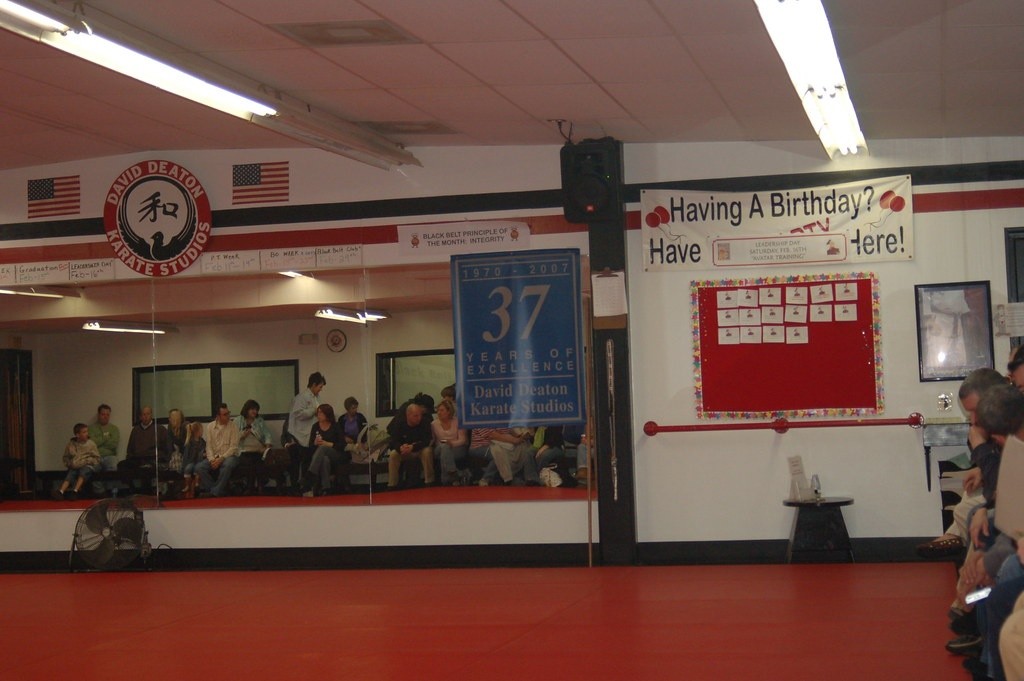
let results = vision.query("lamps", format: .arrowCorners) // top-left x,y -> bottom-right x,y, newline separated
81,320 -> 180,334
313,306 -> 391,324
0,285 -> 82,298
0,0 -> 423,170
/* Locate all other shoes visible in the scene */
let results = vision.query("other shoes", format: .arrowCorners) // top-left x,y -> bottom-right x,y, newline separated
525,479 -> 543,487
52,488 -> 62,502
199,492 -> 213,498
917,534 -> 962,558
946,634 -> 984,653
69,489 -> 78,500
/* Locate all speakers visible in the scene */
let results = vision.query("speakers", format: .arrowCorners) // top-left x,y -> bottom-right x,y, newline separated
560,136 -> 626,223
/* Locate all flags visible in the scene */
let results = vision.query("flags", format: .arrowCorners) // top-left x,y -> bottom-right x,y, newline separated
27,175 -> 81,219
231,161 -> 289,205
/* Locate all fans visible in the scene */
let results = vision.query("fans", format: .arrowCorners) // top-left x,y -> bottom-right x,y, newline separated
69,498 -> 153,572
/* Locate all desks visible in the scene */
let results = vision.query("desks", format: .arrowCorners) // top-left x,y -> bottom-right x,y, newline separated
784,497 -> 855,563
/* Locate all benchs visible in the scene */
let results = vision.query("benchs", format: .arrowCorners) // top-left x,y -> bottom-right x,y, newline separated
49,455 -> 420,501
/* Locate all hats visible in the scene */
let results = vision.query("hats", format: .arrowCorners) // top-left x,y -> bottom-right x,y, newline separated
409,393 -> 435,413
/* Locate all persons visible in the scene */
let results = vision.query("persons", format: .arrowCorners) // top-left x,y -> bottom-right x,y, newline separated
164,403 -> 240,499
469,427 -> 593,487
234,399 -> 273,496
117,407 -> 166,499
87,404 -> 120,493
916,343 -> 1024,681
281,372 -> 368,496
52,423 -> 99,500
386,383 -> 467,489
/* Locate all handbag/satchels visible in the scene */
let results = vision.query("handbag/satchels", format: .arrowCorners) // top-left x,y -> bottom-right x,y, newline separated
541,468 -> 562,488
